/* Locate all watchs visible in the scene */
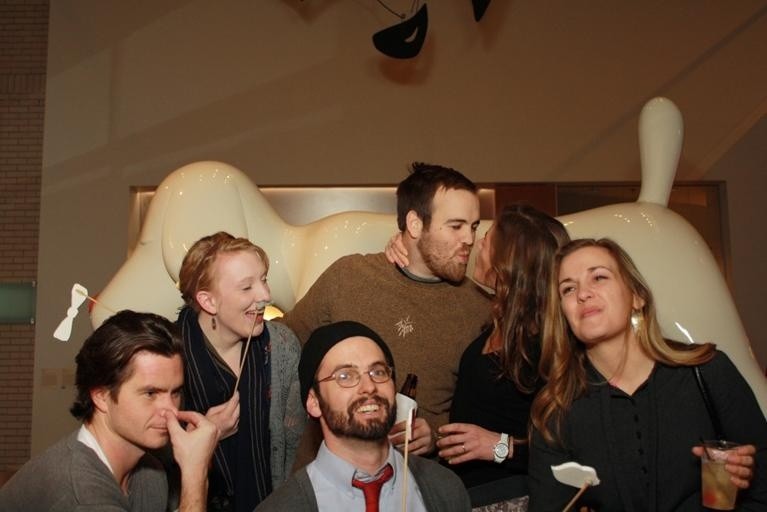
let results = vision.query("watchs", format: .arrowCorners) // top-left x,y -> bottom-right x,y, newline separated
494,432 -> 510,464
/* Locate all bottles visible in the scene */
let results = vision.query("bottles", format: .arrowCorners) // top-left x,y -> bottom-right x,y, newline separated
387,372 -> 420,431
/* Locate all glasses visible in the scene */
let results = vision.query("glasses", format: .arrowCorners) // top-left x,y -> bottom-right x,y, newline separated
314,364 -> 394,388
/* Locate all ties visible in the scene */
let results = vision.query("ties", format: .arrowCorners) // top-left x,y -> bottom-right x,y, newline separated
352,465 -> 393,512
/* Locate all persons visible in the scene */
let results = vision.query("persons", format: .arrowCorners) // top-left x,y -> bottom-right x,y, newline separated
281,161 -> 495,456
172,230 -> 297,512
0,310 -> 221,512
253,322 -> 471,512
526,238 -> 765,511
392,204 -> 572,506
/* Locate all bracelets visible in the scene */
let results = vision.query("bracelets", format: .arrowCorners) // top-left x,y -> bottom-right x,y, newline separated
509,436 -> 514,460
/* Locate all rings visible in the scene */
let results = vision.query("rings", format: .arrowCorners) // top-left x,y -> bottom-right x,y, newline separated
462,446 -> 466,453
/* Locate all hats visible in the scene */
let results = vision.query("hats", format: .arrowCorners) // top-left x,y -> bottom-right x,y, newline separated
298,321 -> 394,409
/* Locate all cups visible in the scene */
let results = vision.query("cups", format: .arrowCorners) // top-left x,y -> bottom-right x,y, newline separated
697,438 -> 740,510
429,415 -> 461,460
